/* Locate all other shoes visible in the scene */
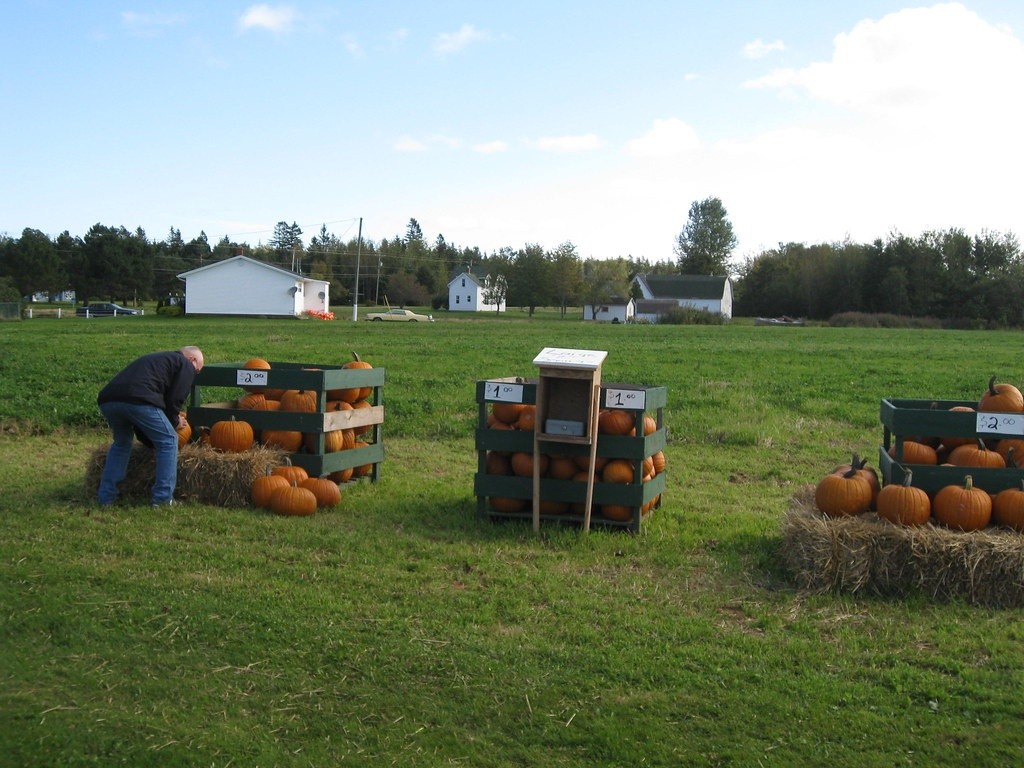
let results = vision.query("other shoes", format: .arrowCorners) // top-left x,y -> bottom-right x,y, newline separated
153,496 -> 188,512
97,503 -> 128,513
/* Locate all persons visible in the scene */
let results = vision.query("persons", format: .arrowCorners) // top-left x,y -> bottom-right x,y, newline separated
97,345 -> 204,510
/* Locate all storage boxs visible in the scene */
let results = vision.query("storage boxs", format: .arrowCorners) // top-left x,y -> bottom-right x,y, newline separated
186,361 -> 386,491
879,398 -> 1024,495
474,377 -> 667,534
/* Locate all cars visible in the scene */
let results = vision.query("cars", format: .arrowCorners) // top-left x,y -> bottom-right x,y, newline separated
363,309 -> 435,323
75,302 -> 144,317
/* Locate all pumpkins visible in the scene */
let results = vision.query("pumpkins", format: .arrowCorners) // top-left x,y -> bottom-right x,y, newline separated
814,376 -> 1024,534
174,351 -> 376,516
485,400 -> 665,522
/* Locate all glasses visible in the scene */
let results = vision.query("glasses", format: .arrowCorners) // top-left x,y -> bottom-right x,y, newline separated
189,357 -> 200,376
